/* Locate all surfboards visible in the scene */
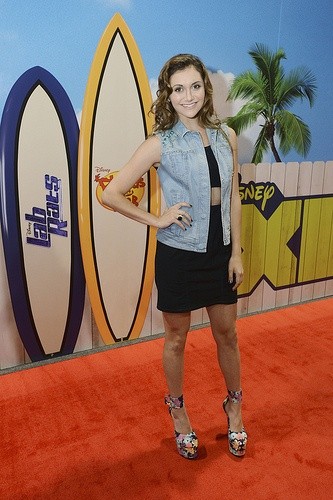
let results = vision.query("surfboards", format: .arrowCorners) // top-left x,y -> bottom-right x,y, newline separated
76,10 -> 162,346
0,65 -> 86,363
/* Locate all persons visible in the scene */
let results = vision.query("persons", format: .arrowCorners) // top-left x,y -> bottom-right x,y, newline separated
101,53 -> 247,458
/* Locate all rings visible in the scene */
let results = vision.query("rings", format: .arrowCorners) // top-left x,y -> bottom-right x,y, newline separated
177,214 -> 182,220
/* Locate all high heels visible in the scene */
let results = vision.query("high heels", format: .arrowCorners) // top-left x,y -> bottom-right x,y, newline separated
163,393 -> 200,459
223,389 -> 248,456
